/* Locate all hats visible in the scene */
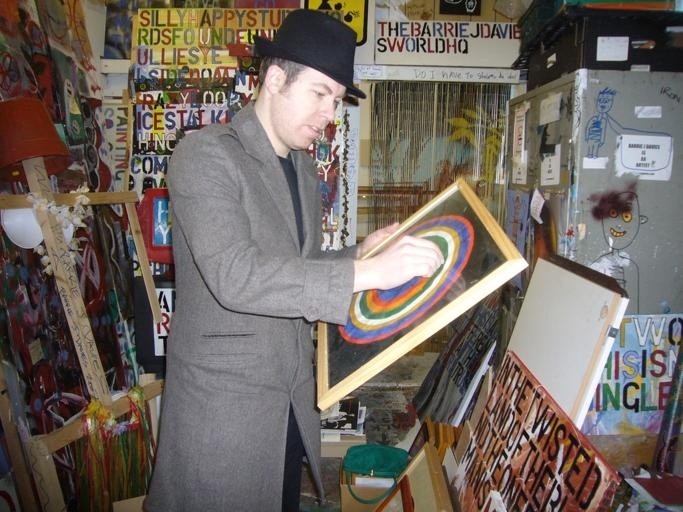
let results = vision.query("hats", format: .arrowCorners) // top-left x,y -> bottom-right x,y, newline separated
252,8 -> 367,99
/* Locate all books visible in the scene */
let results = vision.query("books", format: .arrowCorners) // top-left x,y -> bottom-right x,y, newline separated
319,397 -> 367,437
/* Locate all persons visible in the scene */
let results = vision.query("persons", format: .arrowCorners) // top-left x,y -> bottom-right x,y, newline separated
141,7 -> 446,512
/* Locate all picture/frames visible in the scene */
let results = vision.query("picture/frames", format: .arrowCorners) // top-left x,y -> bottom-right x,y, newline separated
310,178 -> 528,411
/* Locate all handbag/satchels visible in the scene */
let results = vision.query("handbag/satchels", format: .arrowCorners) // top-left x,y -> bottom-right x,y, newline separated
342,443 -> 409,504
